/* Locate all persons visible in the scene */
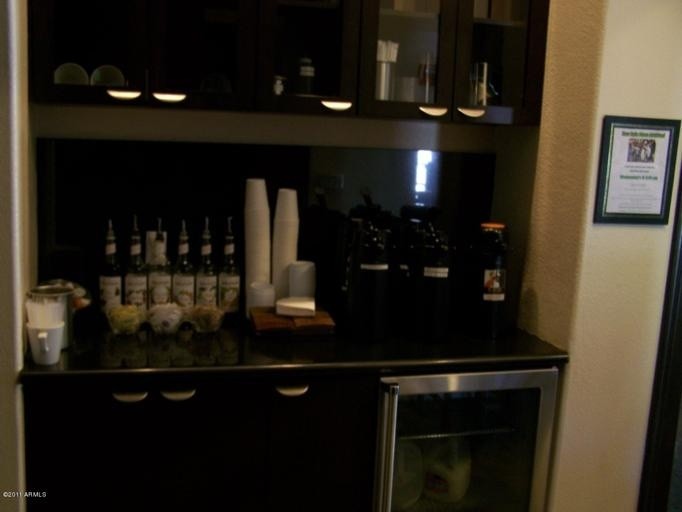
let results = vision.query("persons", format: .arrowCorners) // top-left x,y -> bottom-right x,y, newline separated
628,138 -> 655,162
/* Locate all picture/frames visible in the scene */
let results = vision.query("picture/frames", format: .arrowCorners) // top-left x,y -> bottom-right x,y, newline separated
593,115 -> 682,225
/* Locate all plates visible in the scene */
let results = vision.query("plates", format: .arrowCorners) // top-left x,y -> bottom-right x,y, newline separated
28,0 -> 257,110
257,0 -> 361,116
357,0 -> 548,125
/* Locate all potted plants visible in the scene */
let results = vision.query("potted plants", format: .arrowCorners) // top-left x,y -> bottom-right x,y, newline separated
593,115 -> 682,225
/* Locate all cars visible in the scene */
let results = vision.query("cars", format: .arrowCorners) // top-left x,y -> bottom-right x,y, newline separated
244,177 -> 302,319
54,63 -> 89,85
91,66 -> 129,86
375,60 -> 487,106
25,286 -> 75,365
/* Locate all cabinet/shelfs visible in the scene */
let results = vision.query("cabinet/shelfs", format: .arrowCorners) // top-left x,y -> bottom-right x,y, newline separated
299,58 -> 315,94
24,367 -> 378,512
28,0 -> 257,110
100,236 -> 243,336
257,0 -> 361,116
476,222 -> 509,310
390,439 -> 471,510
357,0 -> 548,125
